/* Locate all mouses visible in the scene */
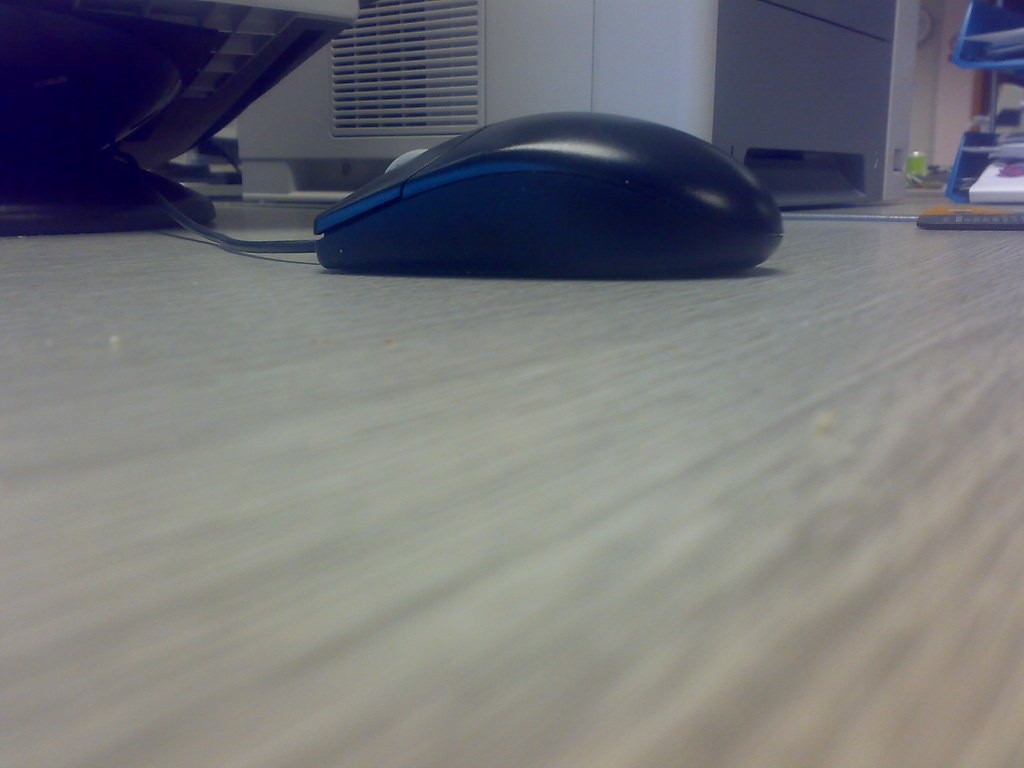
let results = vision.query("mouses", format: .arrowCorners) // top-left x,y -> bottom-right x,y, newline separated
310,112 -> 784,281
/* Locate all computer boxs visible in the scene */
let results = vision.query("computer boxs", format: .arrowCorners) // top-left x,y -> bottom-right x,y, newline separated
238,0 -> 925,207
